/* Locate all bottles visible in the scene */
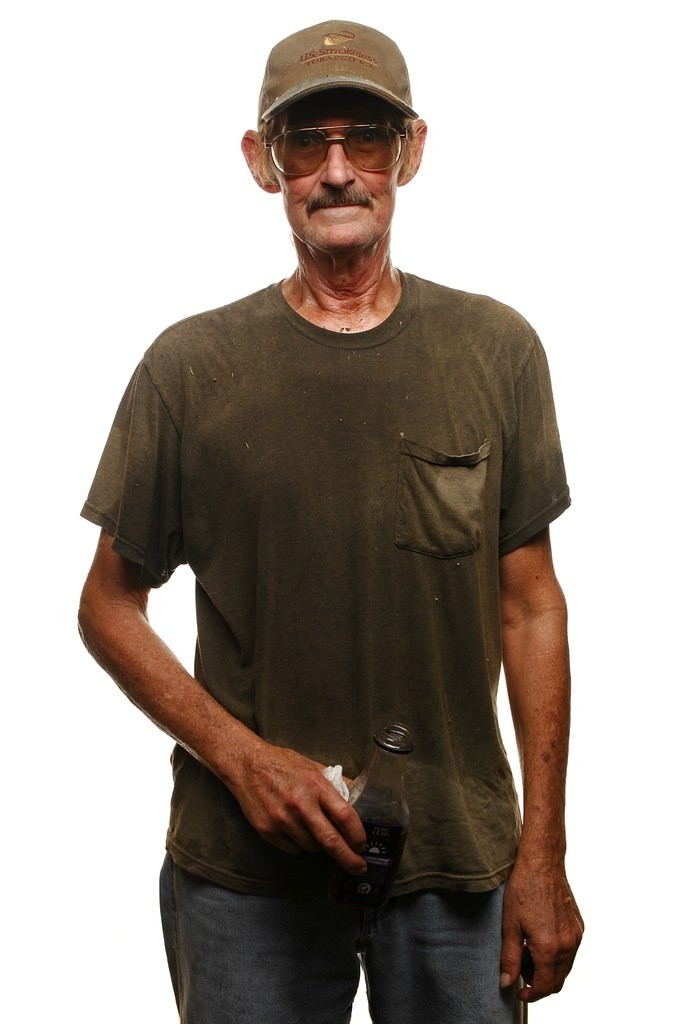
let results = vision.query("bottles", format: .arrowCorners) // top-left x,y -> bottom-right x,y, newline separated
320,722 -> 416,910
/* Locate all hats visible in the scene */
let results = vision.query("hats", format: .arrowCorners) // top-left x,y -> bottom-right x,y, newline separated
258,20 -> 419,125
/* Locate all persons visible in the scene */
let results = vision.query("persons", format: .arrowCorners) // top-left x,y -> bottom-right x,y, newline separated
78,20 -> 586,1024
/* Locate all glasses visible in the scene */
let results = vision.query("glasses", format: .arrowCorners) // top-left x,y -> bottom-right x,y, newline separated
262,123 -> 410,177
